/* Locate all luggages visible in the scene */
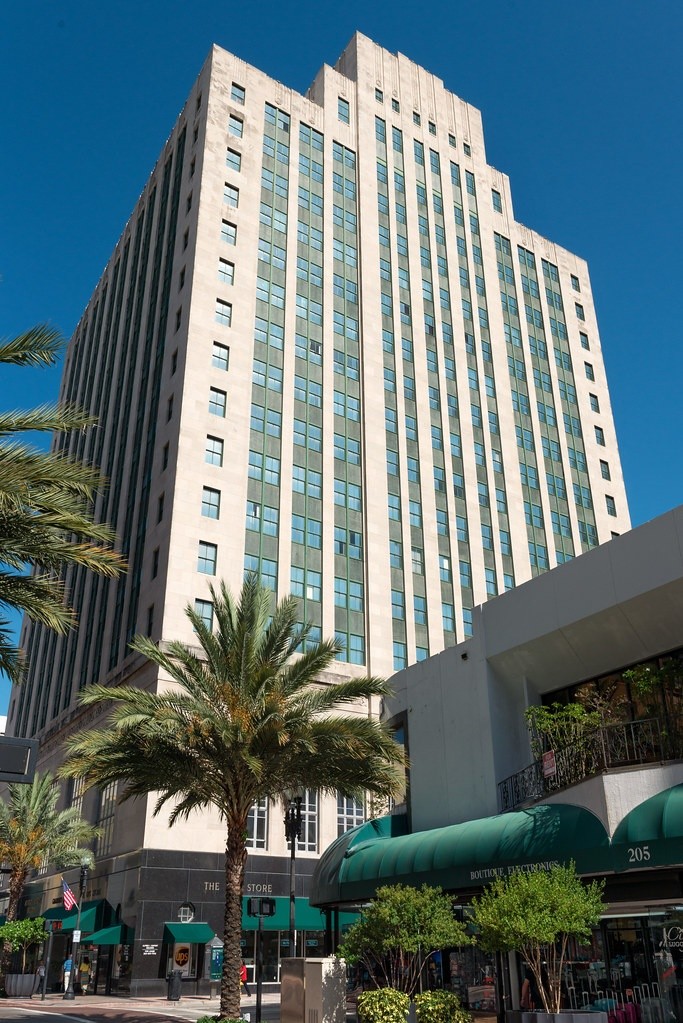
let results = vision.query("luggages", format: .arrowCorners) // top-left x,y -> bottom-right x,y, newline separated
568,983 -> 668,1023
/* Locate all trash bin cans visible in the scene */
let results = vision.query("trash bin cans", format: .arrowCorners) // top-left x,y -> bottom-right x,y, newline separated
166,969 -> 183,1001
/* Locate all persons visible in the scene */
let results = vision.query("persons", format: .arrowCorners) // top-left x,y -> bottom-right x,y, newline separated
30,954 -> 92,999
520,955 -> 554,1011
240,959 -> 252,997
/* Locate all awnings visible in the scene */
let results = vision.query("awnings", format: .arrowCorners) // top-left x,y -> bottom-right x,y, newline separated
41,905 -> 215,945
240,783 -> 682,931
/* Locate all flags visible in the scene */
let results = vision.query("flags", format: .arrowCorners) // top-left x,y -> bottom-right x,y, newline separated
61,878 -> 76,910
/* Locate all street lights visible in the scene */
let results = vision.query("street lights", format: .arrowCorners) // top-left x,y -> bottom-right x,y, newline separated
63,856 -> 92,1000
282,785 -> 303,957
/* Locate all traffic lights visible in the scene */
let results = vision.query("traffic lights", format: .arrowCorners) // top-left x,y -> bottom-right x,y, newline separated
246,895 -> 276,916
